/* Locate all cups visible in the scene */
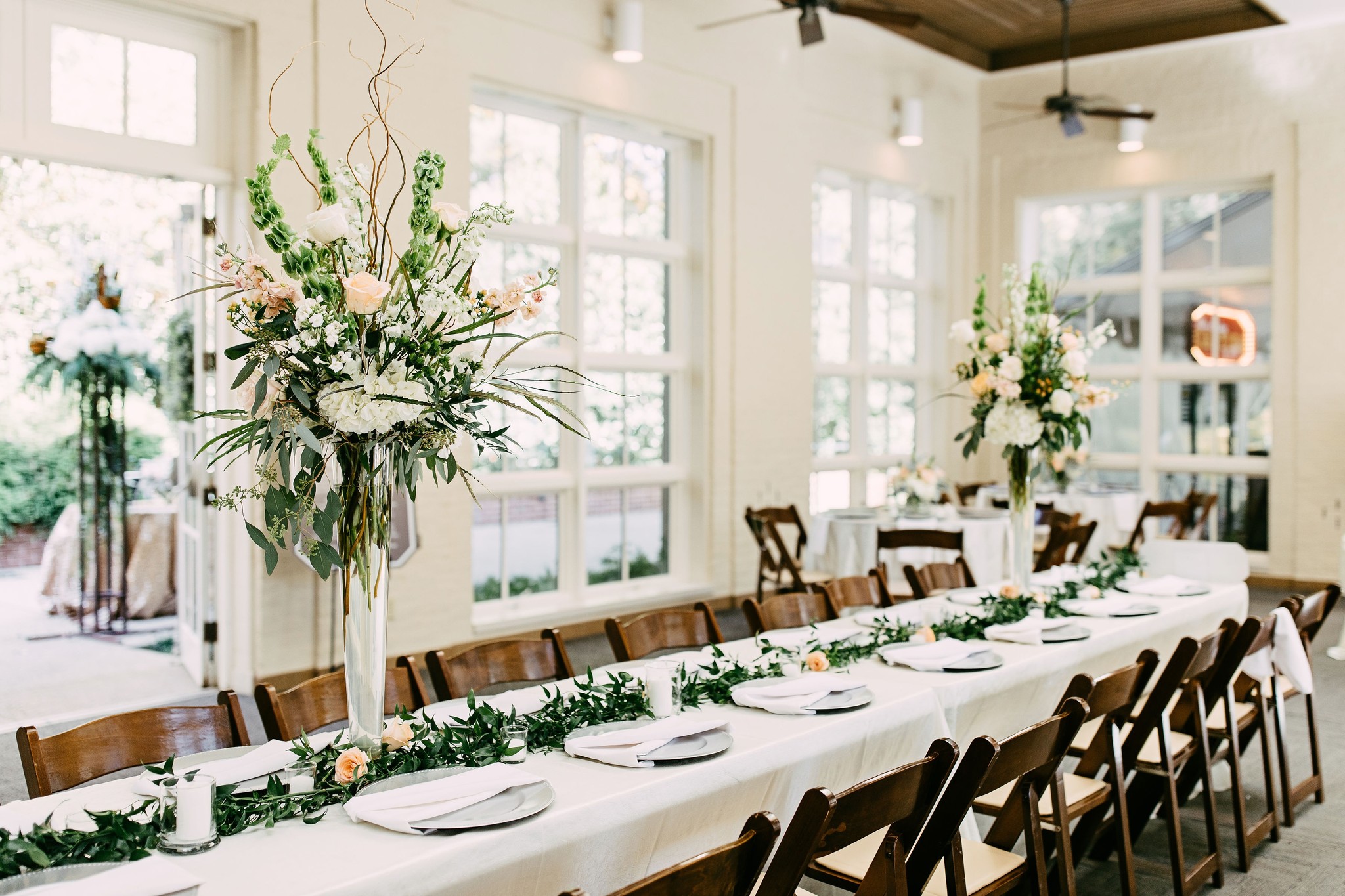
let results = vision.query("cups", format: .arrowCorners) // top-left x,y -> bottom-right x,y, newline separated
160,773 -> 221,855
282,760 -> 317,795
644,659 -> 685,720
1028,602 -> 1046,621
780,653 -> 803,677
499,724 -> 529,766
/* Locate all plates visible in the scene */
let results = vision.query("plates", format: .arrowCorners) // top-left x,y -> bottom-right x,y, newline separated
561,717 -> 732,762
879,640 -> 1002,670
1058,598 -> 1158,615
1,861 -> 199,896
1040,622 -> 1089,644
727,673 -> 873,711
353,765 -> 553,830
1112,573 -> 1207,596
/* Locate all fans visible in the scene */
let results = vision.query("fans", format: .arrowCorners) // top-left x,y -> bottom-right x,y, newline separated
698,4 -> 941,56
993,3 -> 1159,132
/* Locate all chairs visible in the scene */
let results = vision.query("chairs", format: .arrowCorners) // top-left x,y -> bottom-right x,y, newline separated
1024,473 -> 1229,568
965,642 -> 1160,896
1059,614 -> 1245,891
557,800 -> 785,896
789,693 -> 1096,896
1231,579 -> 1344,829
742,740 -> 960,896
1121,594 -> 1297,874
11,512 -> 967,825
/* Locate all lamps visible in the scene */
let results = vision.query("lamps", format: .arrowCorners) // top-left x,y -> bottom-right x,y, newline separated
606,2 -> 648,60
898,94 -> 927,151
1110,98 -> 1146,152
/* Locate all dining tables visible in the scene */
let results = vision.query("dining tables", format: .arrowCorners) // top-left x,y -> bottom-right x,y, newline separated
0,564 -> 1251,895
816,484 -> 1143,598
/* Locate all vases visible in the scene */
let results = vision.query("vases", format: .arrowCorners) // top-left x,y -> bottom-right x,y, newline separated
166,16 -> 602,774
934,256 -> 1129,589
